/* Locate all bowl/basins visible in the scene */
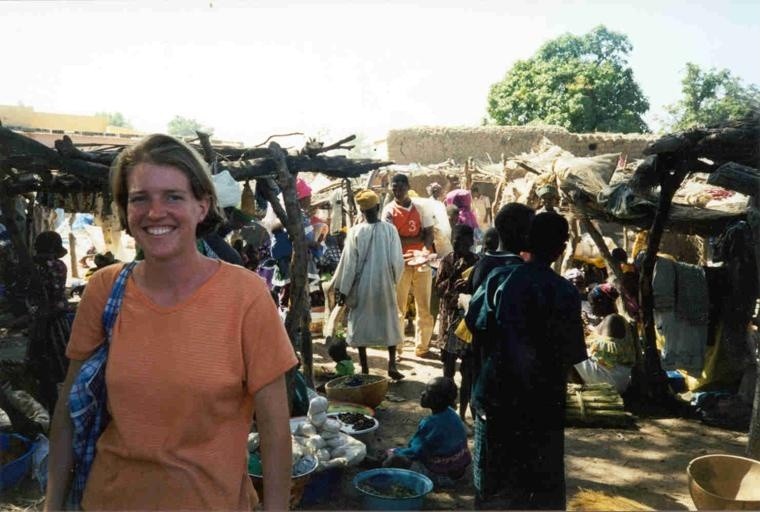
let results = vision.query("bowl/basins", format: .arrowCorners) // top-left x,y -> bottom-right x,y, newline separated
684,454 -> 760,511
326,373 -> 389,410
242,453 -> 319,507
0,428 -> 38,492
326,409 -> 379,446
351,467 -> 436,510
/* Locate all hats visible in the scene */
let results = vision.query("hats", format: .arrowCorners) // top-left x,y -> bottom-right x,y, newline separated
591,281 -> 620,306
354,189 -> 381,213
294,176 -> 317,200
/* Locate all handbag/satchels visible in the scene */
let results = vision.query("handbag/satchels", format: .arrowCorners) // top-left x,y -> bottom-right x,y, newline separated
56,259 -> 142,512
342,271 -> 362,309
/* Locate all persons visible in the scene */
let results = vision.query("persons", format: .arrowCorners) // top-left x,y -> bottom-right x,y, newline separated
42,135 -> 297,510
382,375 -> 471,488
318,227 -> 352,362
611,247 -> 640,298
380,173 -> 436,359
36,230 -> 68,308
196,207 -> 271,271
331,188 -> 405,380
425,183 -> 442,200
569,283 -> 637,394
271,178 -> 328,308
454,212 -> 589,510
467,202 -> 536,295
535,185 -> 562,274
444,185 -> 492,229
433,226 -> 479,421
